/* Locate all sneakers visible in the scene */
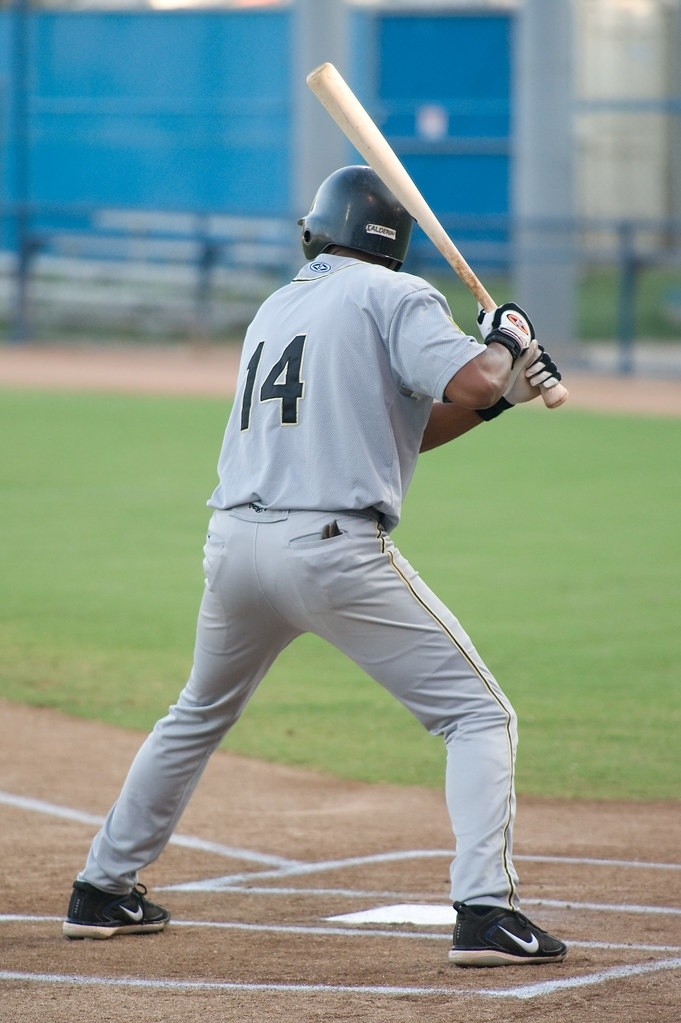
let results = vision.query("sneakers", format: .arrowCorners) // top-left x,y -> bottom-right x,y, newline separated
449,902 -> 568,968
62,878 -> 171,940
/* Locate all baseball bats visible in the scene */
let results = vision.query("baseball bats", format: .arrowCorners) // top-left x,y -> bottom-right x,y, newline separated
305,63 -> 569,409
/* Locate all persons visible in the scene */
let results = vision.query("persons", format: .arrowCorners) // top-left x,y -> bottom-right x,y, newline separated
62,164 -> 568,967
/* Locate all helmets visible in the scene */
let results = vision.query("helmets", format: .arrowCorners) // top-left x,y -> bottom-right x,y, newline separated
296,165 -> 413,274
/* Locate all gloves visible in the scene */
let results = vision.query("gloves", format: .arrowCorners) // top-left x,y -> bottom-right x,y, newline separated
471,340 -> 562,422
475,299 -> 535,368
